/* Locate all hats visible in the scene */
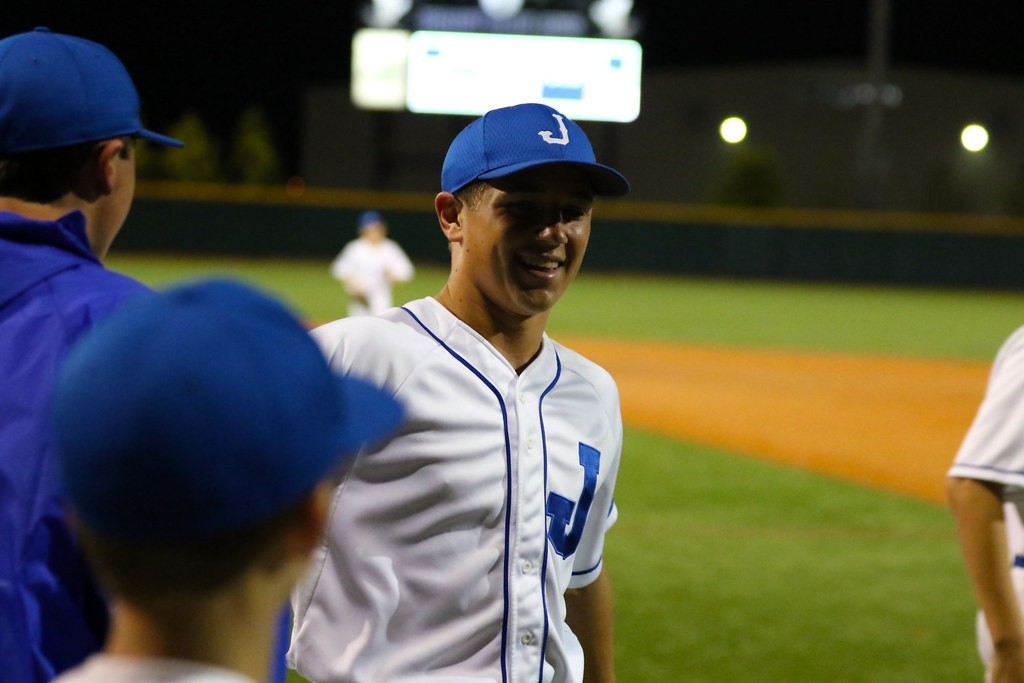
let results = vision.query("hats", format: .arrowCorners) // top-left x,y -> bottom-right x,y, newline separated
440,103 -> 630,196
0,28 -> 182,152
359,212 -> 387,228
59,279 -> 411,594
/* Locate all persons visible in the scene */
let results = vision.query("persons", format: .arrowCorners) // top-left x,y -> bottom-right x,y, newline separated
944,324 -> 1024,682
1,26 -> 296,682
330,208 -> 417,318
52,273 -> 408,682
284,101 -> 625,682
